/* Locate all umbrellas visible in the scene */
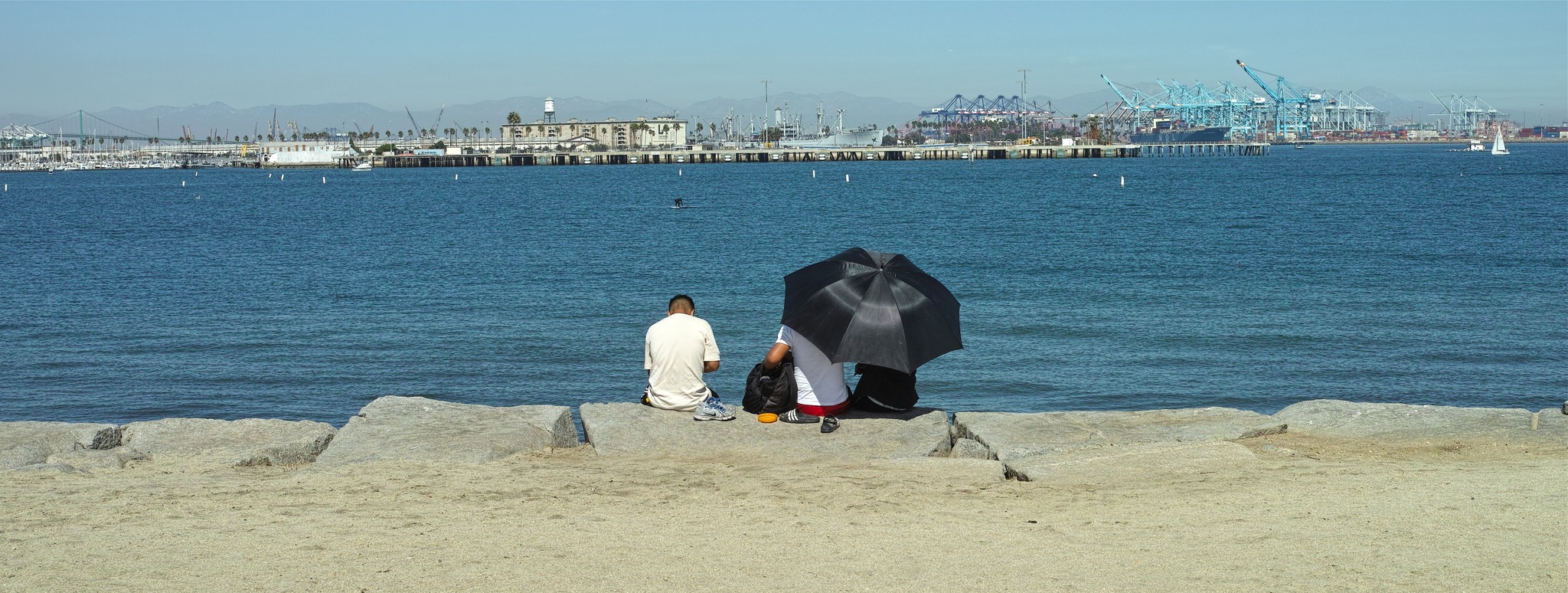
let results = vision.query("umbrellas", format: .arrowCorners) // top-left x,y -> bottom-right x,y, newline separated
779,246 -> 966,377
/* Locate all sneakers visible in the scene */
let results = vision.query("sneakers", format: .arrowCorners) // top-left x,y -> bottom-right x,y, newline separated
693,396 -> 737,420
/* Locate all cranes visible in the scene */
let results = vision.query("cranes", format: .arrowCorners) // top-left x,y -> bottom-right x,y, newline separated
1101,60 -> 1389,140
1428,89 -> 1503,136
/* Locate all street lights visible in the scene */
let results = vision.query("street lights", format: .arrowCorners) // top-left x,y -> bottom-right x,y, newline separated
153,116 -> 162,154
1016,68 -> 1032,139
761,81 -> 772,146
481,121 -> 488,153
691,116 -> 699,150
1539,103 -> 1545,137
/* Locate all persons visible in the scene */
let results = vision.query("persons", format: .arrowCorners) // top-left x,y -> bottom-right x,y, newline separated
640,294 -> 721,412
764,324 -> 854,417
853,363 -> 920,415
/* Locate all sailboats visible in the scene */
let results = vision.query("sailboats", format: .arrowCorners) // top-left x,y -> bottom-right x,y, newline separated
0,126 -> 216,171
1491,126 -> 1510,155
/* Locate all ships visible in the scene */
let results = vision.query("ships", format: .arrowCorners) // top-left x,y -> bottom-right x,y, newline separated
743,101 -> 883,147
1129,120 -> 1232,144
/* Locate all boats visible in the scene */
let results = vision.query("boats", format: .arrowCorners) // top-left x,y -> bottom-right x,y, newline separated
1294,144 -> 1303,149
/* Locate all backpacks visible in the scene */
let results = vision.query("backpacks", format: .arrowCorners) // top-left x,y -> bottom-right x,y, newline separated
742,352 -> 798,415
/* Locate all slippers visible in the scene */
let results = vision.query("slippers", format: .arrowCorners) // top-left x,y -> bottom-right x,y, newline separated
820,414 -> 840,432
780,408 -> 820,423
